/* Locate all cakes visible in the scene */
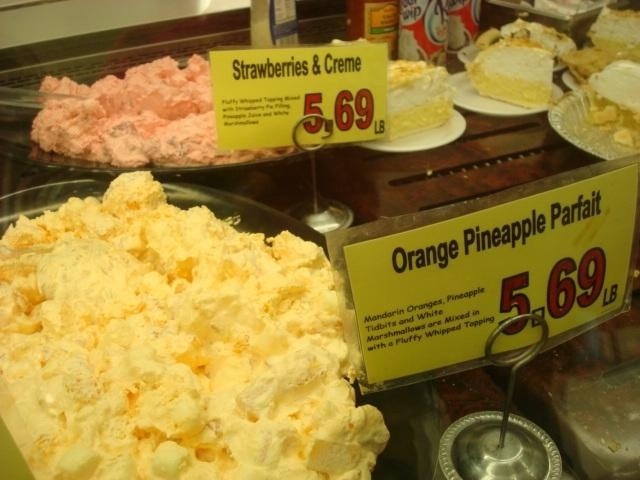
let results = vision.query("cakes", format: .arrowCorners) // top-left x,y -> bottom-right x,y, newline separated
467,6 -> 640,148
329,35 -> 451,143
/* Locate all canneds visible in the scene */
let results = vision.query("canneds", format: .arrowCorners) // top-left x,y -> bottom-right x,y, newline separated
448,1 -> 481,51
344,0 -> 397,59
398,0 -> 448,61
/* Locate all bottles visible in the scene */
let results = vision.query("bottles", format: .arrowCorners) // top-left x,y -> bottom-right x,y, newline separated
341,0 -> 483,69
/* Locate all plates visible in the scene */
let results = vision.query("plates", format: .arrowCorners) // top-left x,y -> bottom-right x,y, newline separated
358,31 -> 634,165
1,133 -> 318,177
1,185 -> 327,253
